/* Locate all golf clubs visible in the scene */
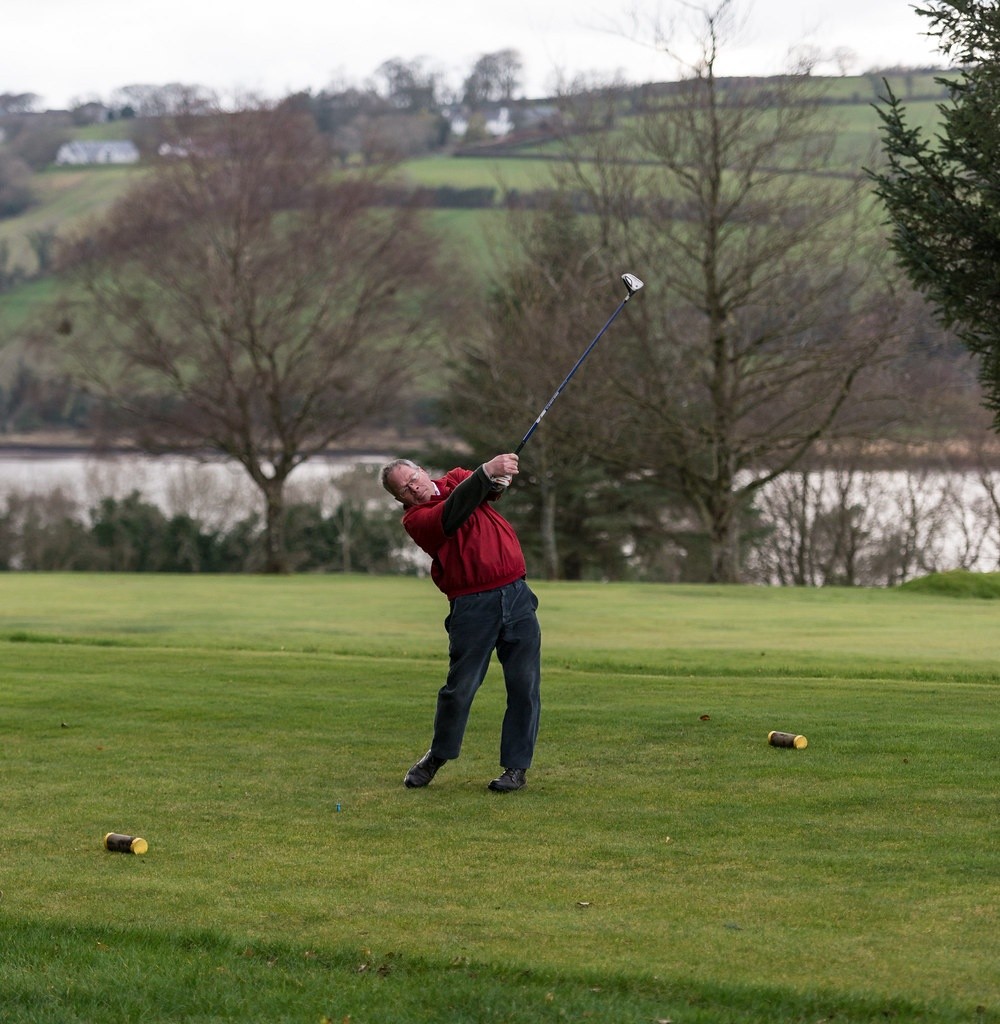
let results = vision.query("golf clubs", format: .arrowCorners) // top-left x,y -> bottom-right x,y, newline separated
513,272 -> 645,456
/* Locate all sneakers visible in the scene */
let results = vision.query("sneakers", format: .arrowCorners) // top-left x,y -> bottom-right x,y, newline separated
488,766 -> 527,792
403,749 -> 448,789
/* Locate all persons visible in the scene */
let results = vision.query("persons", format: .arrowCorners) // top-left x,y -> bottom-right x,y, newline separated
382,453 -> 541,792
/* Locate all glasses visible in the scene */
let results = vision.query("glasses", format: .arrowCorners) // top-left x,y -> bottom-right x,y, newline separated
395,467 -> 421,499
